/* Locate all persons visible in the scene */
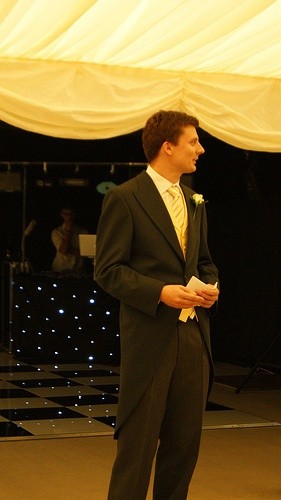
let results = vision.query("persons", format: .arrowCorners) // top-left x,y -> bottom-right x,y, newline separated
92,111 -> 220,500
51,204 -> 92,271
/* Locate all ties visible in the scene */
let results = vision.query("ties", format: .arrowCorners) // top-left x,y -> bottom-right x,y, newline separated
166,185 -> 186,249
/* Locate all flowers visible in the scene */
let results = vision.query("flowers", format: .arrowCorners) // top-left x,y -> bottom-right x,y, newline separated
192,193 -> 205,219
